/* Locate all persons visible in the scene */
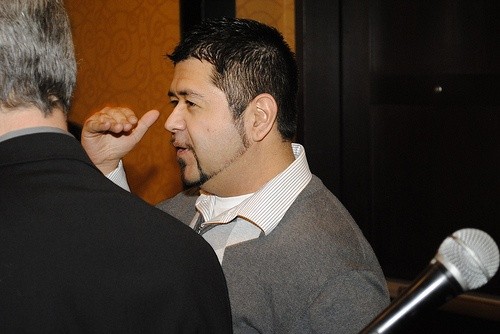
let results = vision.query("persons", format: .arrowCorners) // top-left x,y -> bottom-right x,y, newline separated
0,0 -> 234,334
81,16 -> 393,334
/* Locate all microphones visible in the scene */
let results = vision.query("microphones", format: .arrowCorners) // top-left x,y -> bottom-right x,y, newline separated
355,228 -> 500,334
